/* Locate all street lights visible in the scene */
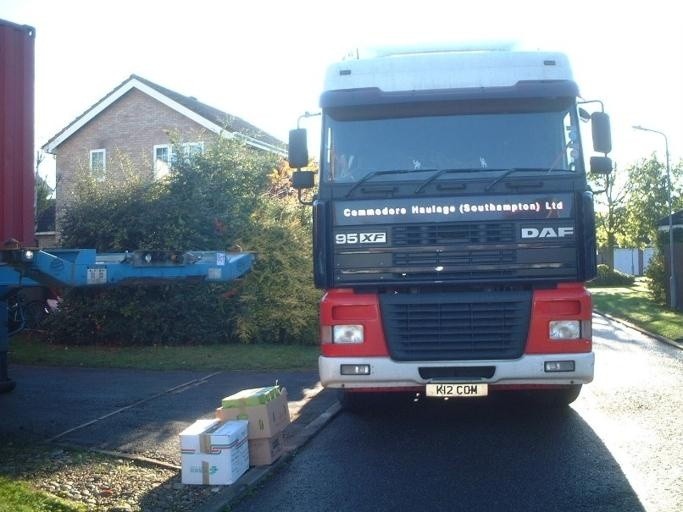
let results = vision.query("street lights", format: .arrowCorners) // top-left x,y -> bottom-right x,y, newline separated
632,125 -> 677,308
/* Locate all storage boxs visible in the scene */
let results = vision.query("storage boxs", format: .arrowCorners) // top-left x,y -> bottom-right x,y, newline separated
180,388 -> 292,484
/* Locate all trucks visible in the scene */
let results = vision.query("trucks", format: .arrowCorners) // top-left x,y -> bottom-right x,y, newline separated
289,39 -> 610,412
